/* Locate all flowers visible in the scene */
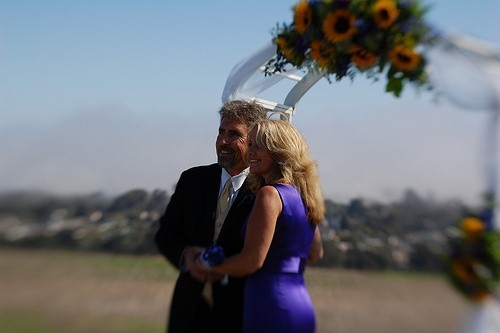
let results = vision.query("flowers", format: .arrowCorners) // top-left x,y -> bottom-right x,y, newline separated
261,0 -> 439,99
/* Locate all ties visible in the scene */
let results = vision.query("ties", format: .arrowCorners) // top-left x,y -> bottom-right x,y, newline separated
214,178 -> 233,244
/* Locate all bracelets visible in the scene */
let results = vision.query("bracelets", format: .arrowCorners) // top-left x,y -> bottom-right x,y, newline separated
192,251 -> 202,265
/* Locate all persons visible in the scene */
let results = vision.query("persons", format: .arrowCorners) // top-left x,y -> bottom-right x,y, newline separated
185,118 -> 324,332
153,101 -> 268,333
